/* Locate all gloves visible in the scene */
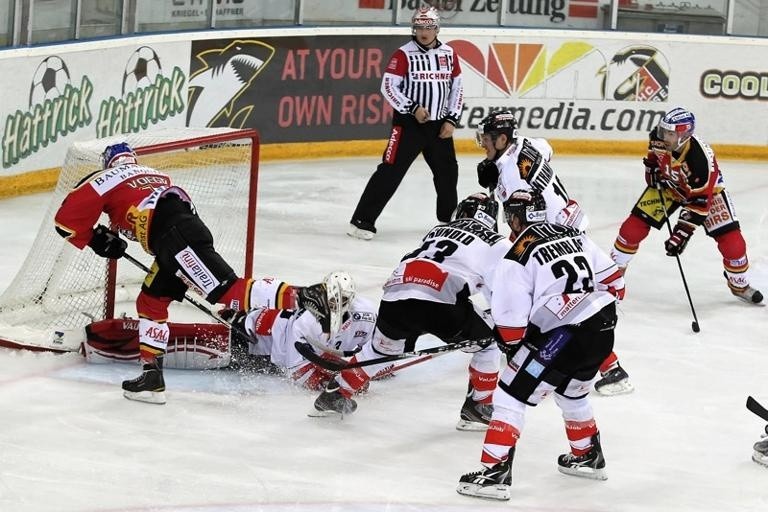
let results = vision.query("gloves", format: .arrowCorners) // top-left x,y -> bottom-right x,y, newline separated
643,157 -> 666,189
665,220 -> 696,256
92,224 -> 128,260
477,159 -> 499,192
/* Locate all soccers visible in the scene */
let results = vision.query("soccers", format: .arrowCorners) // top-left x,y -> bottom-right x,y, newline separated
122,46 -> 162,101
29,55 -> 71,114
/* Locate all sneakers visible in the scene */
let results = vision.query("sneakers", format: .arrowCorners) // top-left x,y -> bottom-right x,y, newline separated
461,384 -> 494,425
295,283 -> 330,333
460,447 -> 515,486
723,270 -> 763,302
314,374 -> 357,414
594,361 -> 629,392
753,441 -> 768,457
350,217 -> 376,233
122,357 -> 165,392
558,431 -> 605,469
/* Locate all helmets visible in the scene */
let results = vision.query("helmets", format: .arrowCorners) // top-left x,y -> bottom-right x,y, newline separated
475,110 -> 519,145
411,6 -> 440,34
503,189 -> 547,226
656,107 -> 695,146
450,193 -> 498,230
322,272 -> 355,316
103,142 -> 138,169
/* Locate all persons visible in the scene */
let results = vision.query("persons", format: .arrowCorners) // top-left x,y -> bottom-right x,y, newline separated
475,111 -> 631,394
86,293 -> 376,399
55,144 -> 343,404
349,4 -> 463,239
610,108 -> 761,304
456,190 -> 617,503
313,193 -> 515,432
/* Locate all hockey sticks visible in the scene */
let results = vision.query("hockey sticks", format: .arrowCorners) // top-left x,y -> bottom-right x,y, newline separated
657,181 -> 700,334
294,335 -> 499,371
745,395 -> 768,423
123,251 -> 259,345
